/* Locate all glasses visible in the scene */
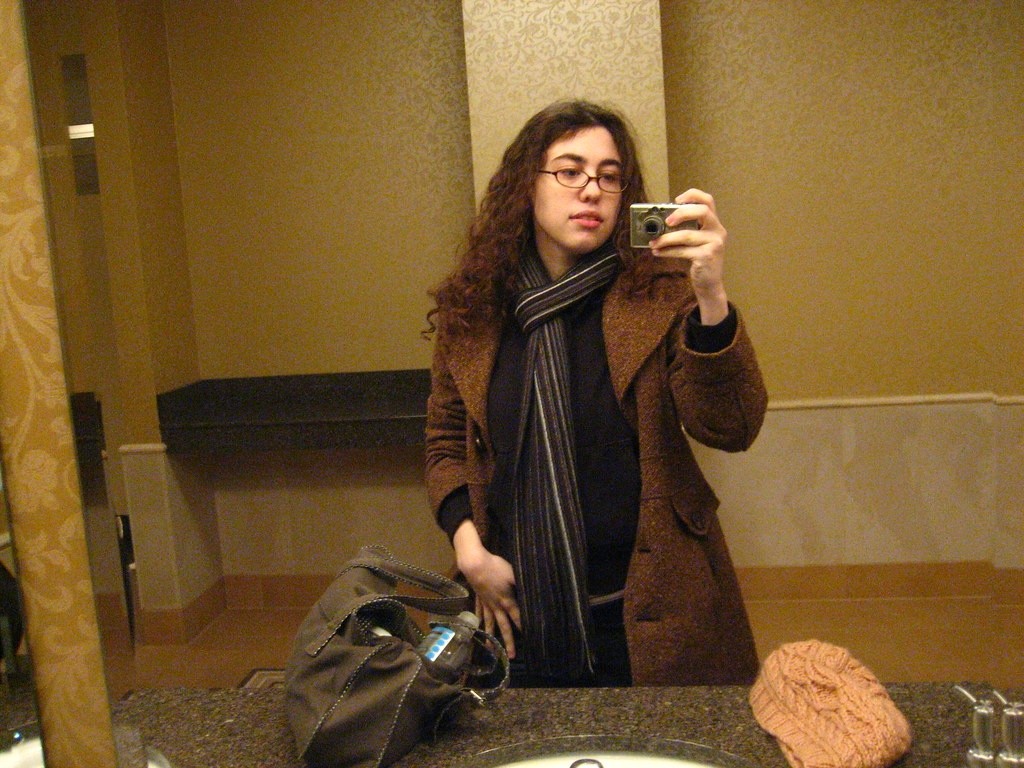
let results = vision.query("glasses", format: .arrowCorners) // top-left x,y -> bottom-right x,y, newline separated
535,168 -> 631,194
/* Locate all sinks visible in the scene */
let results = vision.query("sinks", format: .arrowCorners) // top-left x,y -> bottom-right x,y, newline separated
1,734 -> 170,768
450,732 -> 761,768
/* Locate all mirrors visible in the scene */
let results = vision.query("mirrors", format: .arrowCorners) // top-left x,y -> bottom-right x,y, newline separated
0,0 -> 1024,692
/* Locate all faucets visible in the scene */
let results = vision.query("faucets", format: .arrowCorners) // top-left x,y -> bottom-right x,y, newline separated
569,759 -> 604,766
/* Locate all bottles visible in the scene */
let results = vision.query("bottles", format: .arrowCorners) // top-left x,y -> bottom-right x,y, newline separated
415,610 -> 481,685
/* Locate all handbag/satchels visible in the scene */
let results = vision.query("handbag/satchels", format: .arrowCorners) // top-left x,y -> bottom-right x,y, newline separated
285,544 -> 508,768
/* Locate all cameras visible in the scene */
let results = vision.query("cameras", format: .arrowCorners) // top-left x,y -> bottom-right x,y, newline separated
631,203 -> 699,248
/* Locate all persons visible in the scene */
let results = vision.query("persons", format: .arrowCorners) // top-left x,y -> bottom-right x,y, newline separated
425,99 -> 769,691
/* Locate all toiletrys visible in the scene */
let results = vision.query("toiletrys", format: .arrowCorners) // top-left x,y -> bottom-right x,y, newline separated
955,684 -> 1023,768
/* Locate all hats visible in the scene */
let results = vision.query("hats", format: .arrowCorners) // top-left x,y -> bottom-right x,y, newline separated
748,641 -> 912,768
